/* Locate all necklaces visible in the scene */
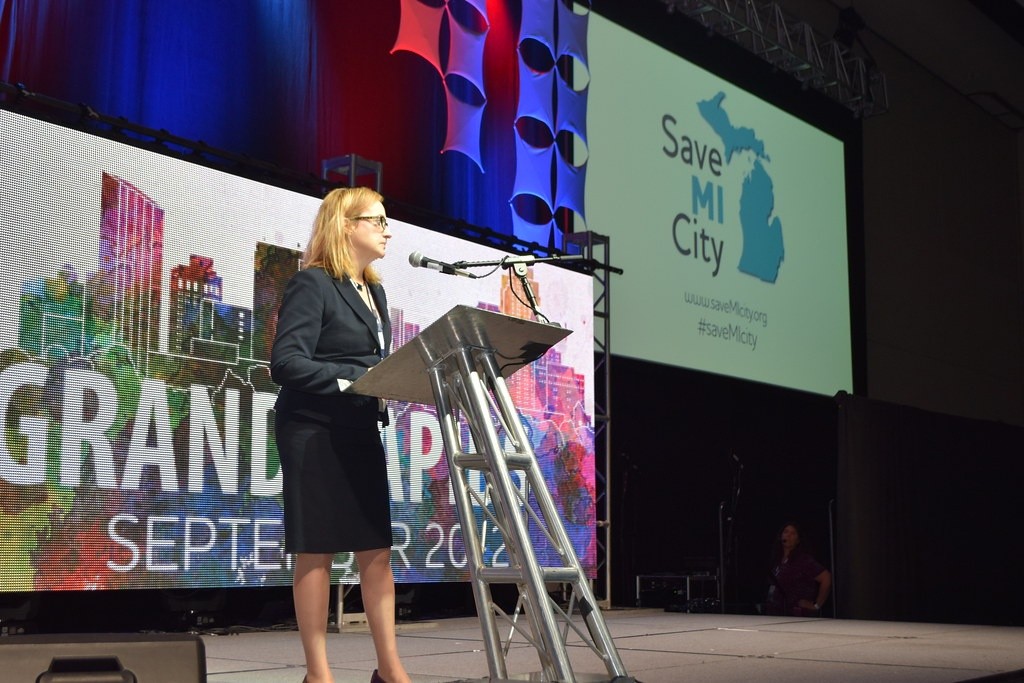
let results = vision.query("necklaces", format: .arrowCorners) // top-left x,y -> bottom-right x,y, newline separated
353,278 -> 364,292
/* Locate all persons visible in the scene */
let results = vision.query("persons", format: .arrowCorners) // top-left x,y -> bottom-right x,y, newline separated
270,187 -> 413,683
768,523 -> 832,617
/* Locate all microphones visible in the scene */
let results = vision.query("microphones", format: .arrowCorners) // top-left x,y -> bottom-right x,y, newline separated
409,251 -> 477,279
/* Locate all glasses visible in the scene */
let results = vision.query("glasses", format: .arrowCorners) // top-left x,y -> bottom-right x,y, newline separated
350,215 -> 388,230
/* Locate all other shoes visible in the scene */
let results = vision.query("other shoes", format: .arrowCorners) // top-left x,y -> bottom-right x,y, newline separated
369,669 -> 385,683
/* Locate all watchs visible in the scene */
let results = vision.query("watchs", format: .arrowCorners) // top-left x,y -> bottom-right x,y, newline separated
813,601 -> 820,608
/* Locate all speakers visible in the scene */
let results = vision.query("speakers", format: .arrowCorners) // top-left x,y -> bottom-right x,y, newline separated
0,633 -> 209,682
